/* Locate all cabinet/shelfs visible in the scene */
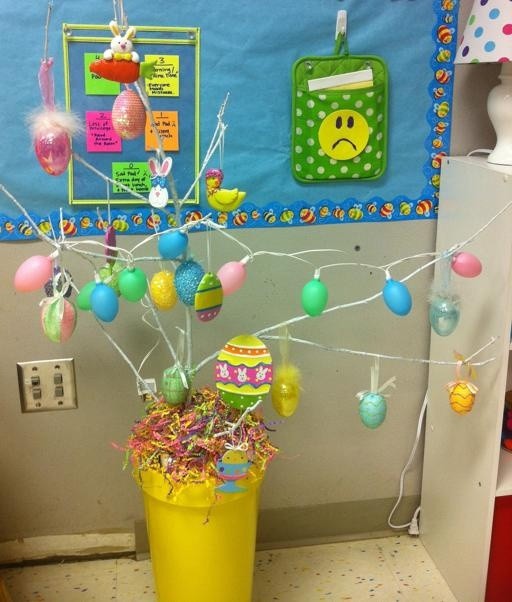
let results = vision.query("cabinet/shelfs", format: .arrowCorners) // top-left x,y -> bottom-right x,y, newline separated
419,155 -> 512,602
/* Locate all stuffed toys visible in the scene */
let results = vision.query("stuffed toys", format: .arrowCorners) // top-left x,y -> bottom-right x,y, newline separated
91,20 -> 162,88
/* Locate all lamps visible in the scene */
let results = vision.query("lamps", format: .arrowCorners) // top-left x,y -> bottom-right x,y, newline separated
453,0 -> 512,166
